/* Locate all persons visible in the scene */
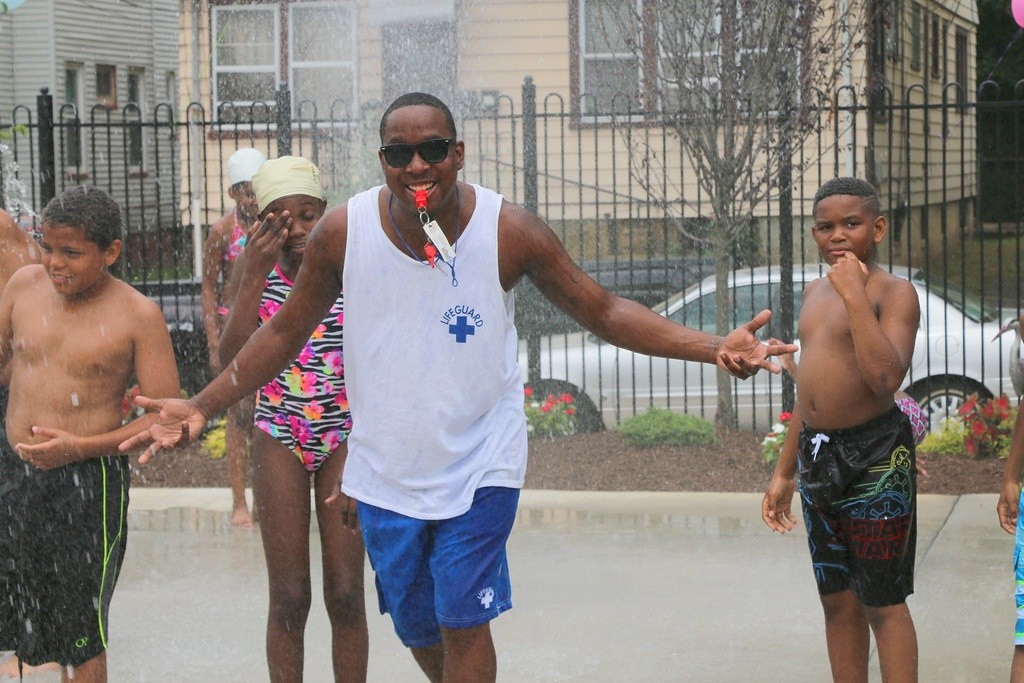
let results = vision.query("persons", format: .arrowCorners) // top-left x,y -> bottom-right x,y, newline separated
996,311 -> 1024,683
118,92 -> 798,683
761,177 -> 927,683
0,185 -> 181,683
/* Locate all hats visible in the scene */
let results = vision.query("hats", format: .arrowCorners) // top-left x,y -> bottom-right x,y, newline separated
227,147 -> 267,187
251,155 -> 327,213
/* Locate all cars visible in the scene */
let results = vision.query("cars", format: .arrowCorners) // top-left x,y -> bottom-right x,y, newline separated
518,263 -> 1024,436
